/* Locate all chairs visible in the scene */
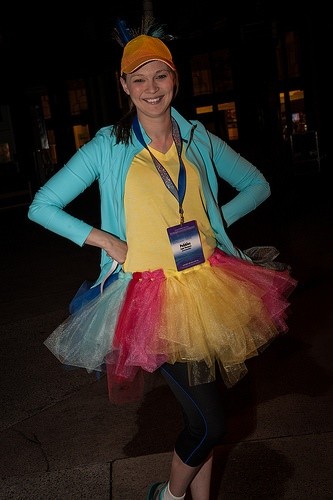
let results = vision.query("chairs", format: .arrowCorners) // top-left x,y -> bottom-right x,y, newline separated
0,161 -> 33,210
289,131 -> 327,170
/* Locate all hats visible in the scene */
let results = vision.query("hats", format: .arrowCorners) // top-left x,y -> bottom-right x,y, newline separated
120,35 -> 176,78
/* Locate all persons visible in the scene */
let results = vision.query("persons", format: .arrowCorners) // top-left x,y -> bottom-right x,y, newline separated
28,36 -> 297,500
